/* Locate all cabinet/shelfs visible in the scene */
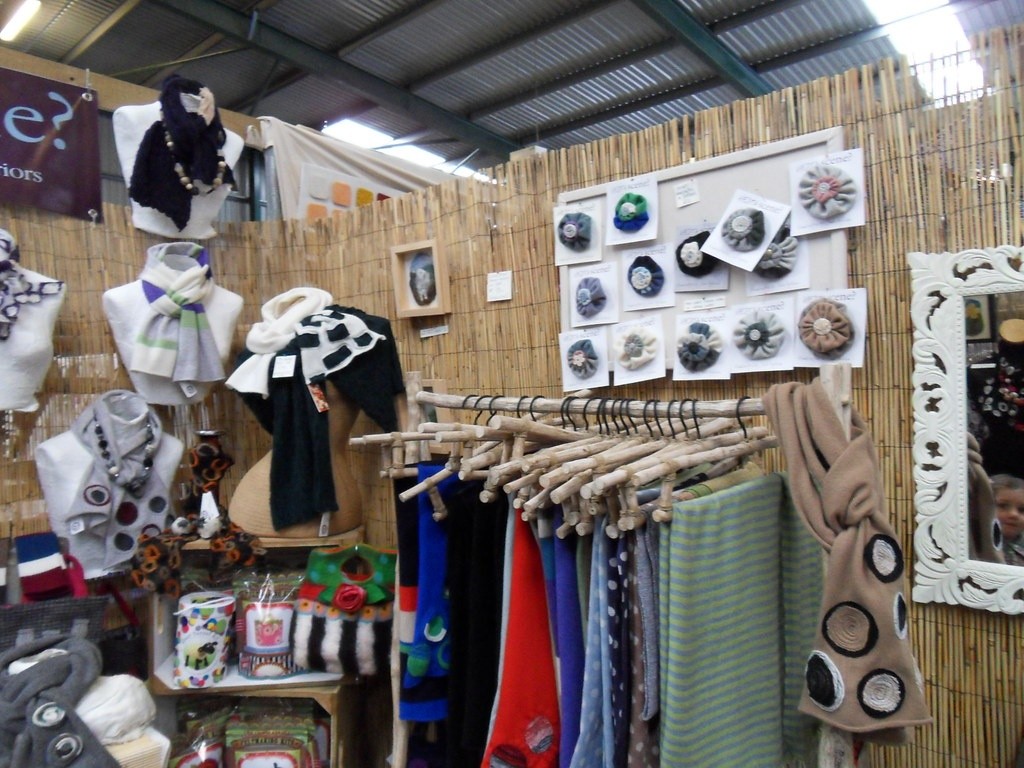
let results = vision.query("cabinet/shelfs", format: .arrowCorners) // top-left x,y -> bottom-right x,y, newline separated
145,525 -> 365,768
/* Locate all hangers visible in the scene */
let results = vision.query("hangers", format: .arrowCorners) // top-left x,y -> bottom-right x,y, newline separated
348,395 -> 780,539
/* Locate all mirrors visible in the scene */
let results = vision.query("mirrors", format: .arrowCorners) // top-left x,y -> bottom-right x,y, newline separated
905,244 -> 1024,616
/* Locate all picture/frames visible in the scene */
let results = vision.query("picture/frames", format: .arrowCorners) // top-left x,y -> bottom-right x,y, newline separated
390,237 -> 452,319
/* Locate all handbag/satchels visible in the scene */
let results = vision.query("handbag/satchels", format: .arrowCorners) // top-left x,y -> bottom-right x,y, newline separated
0,554 -> 106,654
97,581 -> 151,686
291,543 -> 396,675
